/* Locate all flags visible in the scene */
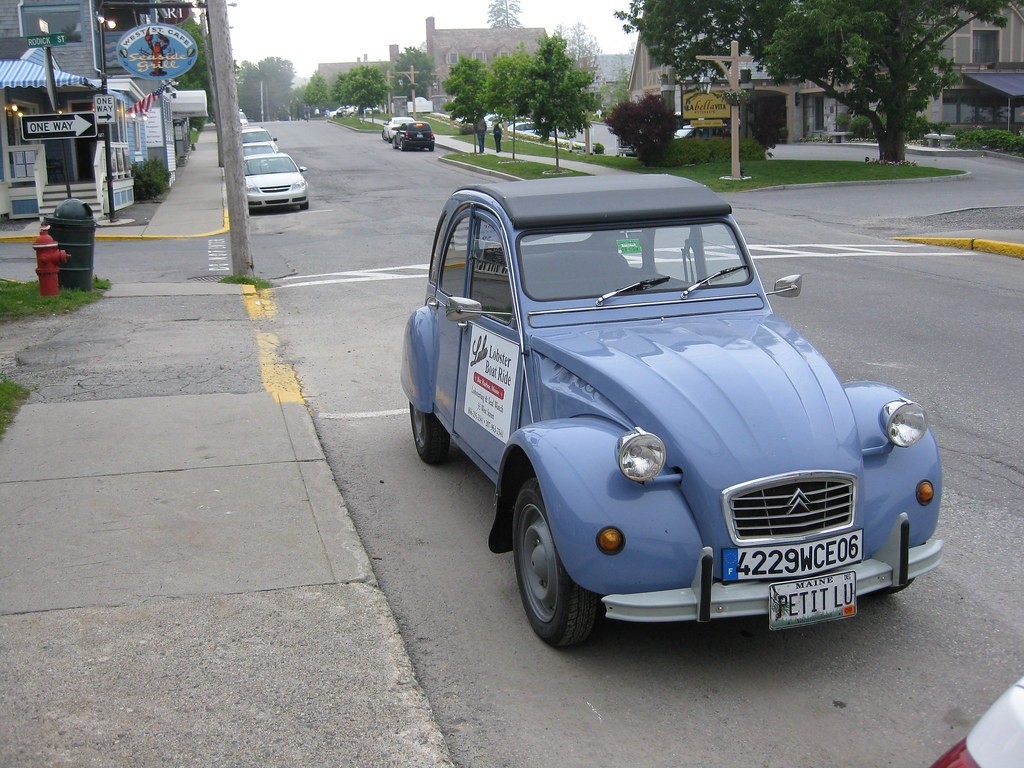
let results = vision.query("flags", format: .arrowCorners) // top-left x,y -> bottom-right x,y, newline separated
126,85 -> 167,114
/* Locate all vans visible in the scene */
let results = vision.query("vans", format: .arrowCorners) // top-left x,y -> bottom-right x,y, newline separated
429,95 -> 449,111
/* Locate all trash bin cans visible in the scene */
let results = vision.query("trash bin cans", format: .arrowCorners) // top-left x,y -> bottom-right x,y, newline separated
43,197 -> 97,293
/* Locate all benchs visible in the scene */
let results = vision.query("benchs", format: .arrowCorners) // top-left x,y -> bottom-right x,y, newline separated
522,251 -> 636,301
823,131 -> 855,143
924,133 -> 957,147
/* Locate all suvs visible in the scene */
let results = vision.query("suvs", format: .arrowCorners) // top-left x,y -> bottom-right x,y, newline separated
329,105 -> 383,120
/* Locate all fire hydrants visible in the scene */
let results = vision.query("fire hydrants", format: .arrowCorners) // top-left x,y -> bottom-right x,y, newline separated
31,222 -> 72,297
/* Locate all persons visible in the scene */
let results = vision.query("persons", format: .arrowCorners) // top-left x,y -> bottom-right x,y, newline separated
493,124 -> 502,153
474,116 -> 487,153
303,105 -> 330,120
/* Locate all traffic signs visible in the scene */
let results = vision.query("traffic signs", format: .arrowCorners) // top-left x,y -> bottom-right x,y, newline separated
93,95 -> 116,125
20,112 -> 98,141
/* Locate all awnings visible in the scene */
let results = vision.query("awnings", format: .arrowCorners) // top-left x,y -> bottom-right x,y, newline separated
960,72 -> 1024,98
0,60 -> 96,90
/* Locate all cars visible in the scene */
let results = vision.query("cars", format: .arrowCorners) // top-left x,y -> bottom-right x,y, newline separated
239,109 -> 249,127
241,142 -> 279,167
673,127 -> 702,139
392,121 -> 435,151
241,125 -> 263,129
381,116 -> 415,143
506,121 -> 538,135
399,174 -> 944,649
243,155 -> 309,214
241,129 -> 280,153
483,114 -> 531,134
